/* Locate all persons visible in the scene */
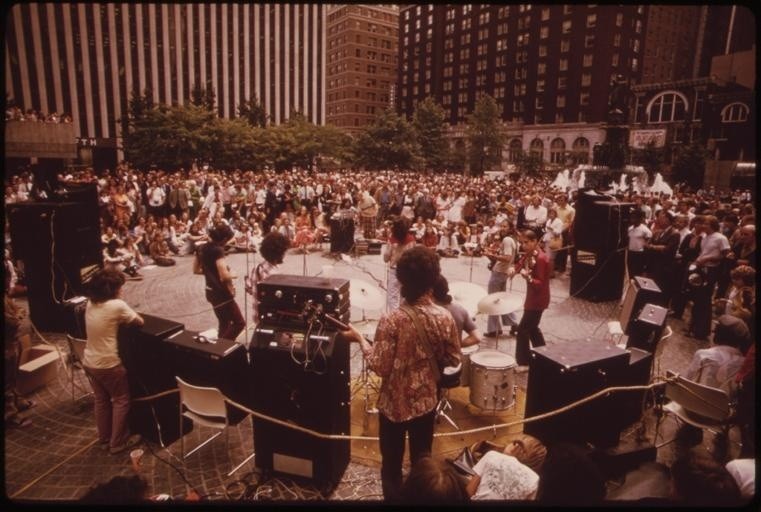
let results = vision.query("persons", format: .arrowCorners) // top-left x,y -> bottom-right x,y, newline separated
466,433 -> 549,500
82,474 -> 172,501
82,267 -> 143,455
3,161 -> 760,429
526,430 -> 611,510
665,455 -> 755,507
386,455 -> 467,501
338,245 -> 465,496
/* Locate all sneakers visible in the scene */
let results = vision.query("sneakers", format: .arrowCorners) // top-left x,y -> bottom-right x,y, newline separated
99,433 -> 111,445
509,324 -> 519,334
6,415 -> 33,428
111,433 -> 143,455
483,329 -> 498,337
17,399 -> 37,413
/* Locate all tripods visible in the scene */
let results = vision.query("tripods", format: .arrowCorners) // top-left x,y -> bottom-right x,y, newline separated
434,393 -> 462,435
352,347 -> 385,415
51,345 -> 93,410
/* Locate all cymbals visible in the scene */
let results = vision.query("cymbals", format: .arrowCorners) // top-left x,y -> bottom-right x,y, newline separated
449,282 -> 487,313
478,291 -> 524,317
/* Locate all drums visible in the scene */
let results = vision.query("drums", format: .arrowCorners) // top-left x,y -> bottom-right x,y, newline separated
468,349 -> 517,413
443,361 -> 465,388
461,343 -> 480,387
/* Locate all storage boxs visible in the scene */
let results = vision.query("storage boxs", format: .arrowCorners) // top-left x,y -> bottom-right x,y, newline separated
14,341 -> 67,389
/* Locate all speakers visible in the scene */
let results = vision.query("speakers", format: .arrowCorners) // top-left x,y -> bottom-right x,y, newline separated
5,199 -> 101,255
249,323 -> 350,496
20,255 -> 109,339
625,302 -> 669,355
522,339 -> 632,452
621,346 -> 654,429
573,187 -> 636,244
331,220 -> 354,251
619,275 -> 663,334
570,246 -> 625,300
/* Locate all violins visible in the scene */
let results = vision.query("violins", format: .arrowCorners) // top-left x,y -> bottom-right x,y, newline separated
520,249 -> 537,285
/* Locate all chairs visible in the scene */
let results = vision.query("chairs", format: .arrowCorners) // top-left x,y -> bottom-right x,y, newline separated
649,368 -> 740,470
64,331 -> 97,374
164,374 -> 259,476
